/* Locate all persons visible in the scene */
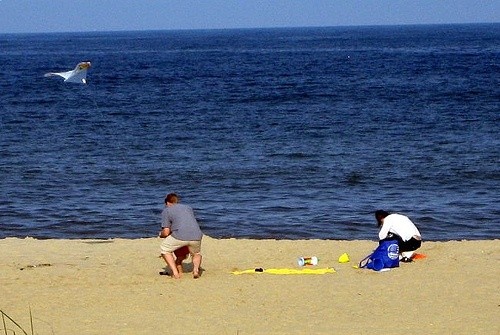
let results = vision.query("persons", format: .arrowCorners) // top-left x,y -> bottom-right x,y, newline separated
158,192 -> 203,279
375,209 -> 422,262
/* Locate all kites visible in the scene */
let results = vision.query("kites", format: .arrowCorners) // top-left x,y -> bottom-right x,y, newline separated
43,62 -> 92,84
